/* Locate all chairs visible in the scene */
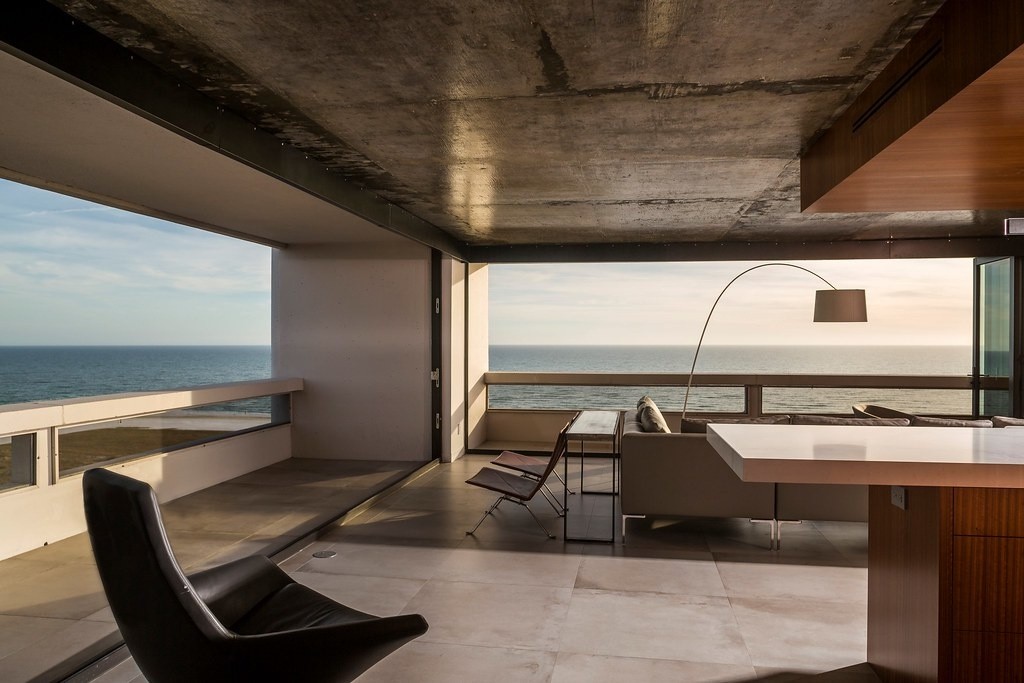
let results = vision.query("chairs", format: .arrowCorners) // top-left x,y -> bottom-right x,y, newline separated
80,467 -> 430,683
463,412 -> 583,540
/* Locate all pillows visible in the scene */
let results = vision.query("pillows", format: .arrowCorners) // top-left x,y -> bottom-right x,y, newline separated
638,396 -> 1023,432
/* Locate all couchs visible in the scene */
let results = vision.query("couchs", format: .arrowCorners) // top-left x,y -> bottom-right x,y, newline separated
620,408 -> 1023,551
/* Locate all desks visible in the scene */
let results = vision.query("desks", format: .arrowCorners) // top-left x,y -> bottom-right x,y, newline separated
563,407 -> 624,544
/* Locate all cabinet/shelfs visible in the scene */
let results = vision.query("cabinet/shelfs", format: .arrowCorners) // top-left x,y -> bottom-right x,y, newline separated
866,483 -> 1023,683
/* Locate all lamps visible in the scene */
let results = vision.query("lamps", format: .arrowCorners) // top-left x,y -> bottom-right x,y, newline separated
681,263 -> 868,422
